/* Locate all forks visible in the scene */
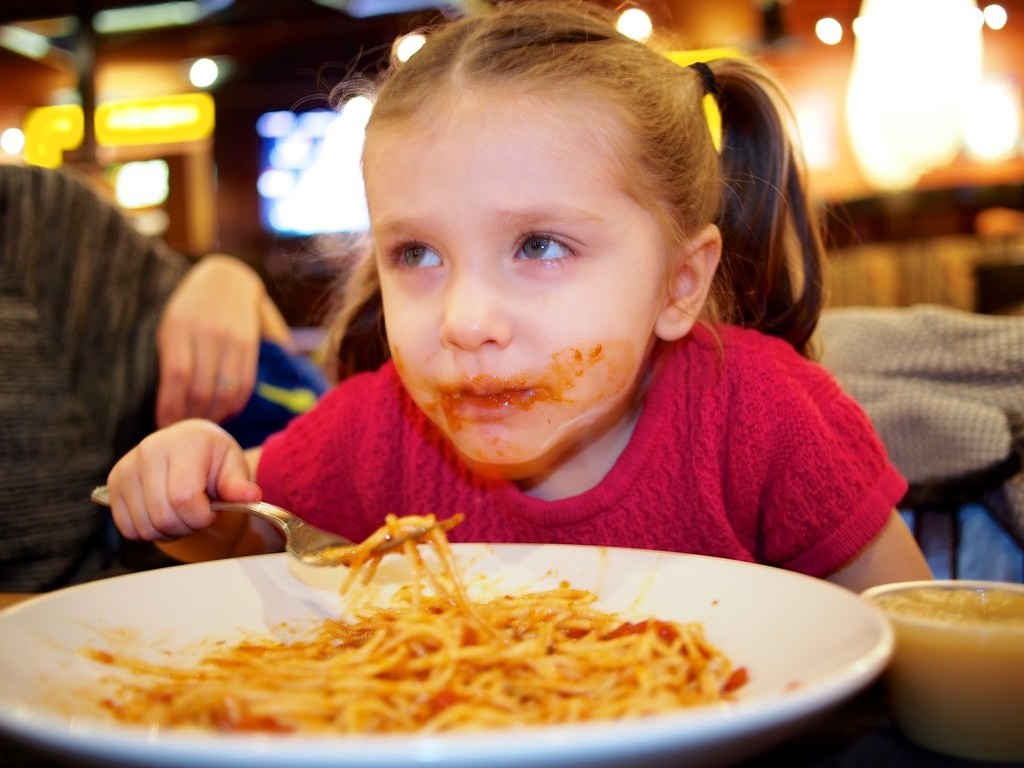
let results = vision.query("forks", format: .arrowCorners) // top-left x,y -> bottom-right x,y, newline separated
90,484 -> 465,567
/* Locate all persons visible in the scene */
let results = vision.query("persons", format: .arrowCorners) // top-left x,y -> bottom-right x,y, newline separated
107,3 -> 935,594
0,164 -> 296,593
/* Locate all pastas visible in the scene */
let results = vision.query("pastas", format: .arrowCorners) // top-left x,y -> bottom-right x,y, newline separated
66,513 -> 748,738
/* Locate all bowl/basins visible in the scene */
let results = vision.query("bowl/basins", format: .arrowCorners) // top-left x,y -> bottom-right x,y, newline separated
859,579 -> 1024,761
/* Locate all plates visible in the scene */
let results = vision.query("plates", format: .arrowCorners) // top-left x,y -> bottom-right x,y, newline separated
0,542 -> 895,768
